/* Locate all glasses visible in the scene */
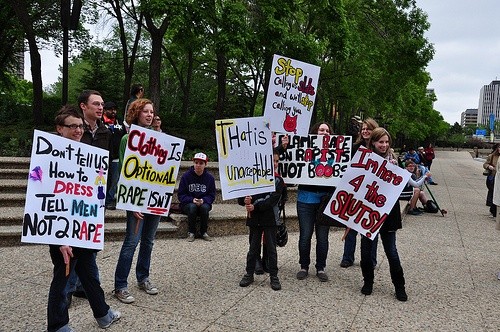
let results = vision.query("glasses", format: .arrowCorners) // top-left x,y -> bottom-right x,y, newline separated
154,119 -> 161,121
61,124 -> 86,130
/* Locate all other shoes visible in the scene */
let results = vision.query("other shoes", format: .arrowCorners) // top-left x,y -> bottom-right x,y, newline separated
240,274 -> 254,287
339,259 -> 353,269
102,311 -> 124,328
409,207 -> 421,215
255,256 -> 271,276
395,286 -> 408,301
361,283 -> 374,295
187,232 -> 195,242
203,232 -> 213,242
315,270 -> 329,282
269,276 -> 281,291
430,181 -> 438,186
296,270 -> 308,280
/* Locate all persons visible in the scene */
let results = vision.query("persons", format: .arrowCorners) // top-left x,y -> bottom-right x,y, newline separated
146,114 -> 163,133
352,127 -> 408,302
237,145 -> 286,293
47,108 -> 123,332
398,142 -> 439,216
62,89 -> 112,300
483,143 -> 500,218
295,120 -> 337,283
100,102 -> 127,211
111,98 -> 159,304
340,118 -> 381,272
177,152 -> 217,243
122,83 -> 145,135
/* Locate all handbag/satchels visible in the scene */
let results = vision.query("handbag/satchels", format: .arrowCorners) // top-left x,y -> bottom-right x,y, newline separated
482,169 -> 489,176
277,222 -> 290,247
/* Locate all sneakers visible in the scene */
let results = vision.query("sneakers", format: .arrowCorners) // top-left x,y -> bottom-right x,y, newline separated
138,279 -> 162,295
114,289 -> 136,303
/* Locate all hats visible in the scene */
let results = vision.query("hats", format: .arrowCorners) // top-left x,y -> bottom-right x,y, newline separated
192,153 -> 209,163
104,101 -> 118,110
